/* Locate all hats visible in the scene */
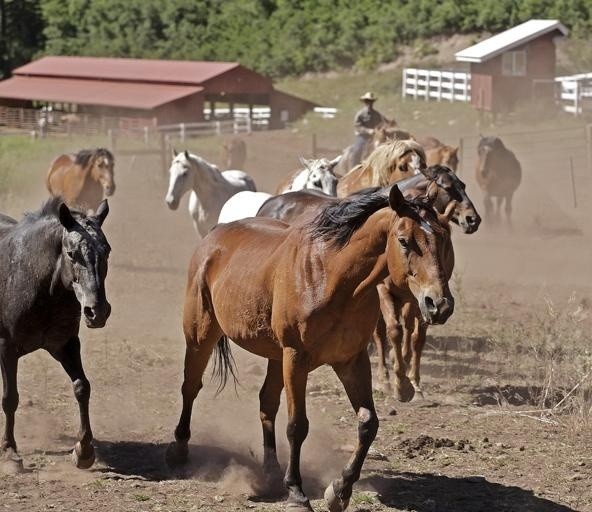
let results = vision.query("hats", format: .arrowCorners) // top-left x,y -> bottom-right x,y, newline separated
360,92 -> 377,102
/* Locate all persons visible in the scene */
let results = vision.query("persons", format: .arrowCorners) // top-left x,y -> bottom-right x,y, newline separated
350,92 -> 390,165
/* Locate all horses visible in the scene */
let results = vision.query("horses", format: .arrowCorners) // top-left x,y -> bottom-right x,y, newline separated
217,152 -> 343,225
336,135 -> 427,203
46,149 -> 115,216
476,135 -> 521,226
164,149 -> 256,242
163,179 -> 455,512
0,196 -> 111,476
256,160 -> 481,402
332,133 -> 459,178
